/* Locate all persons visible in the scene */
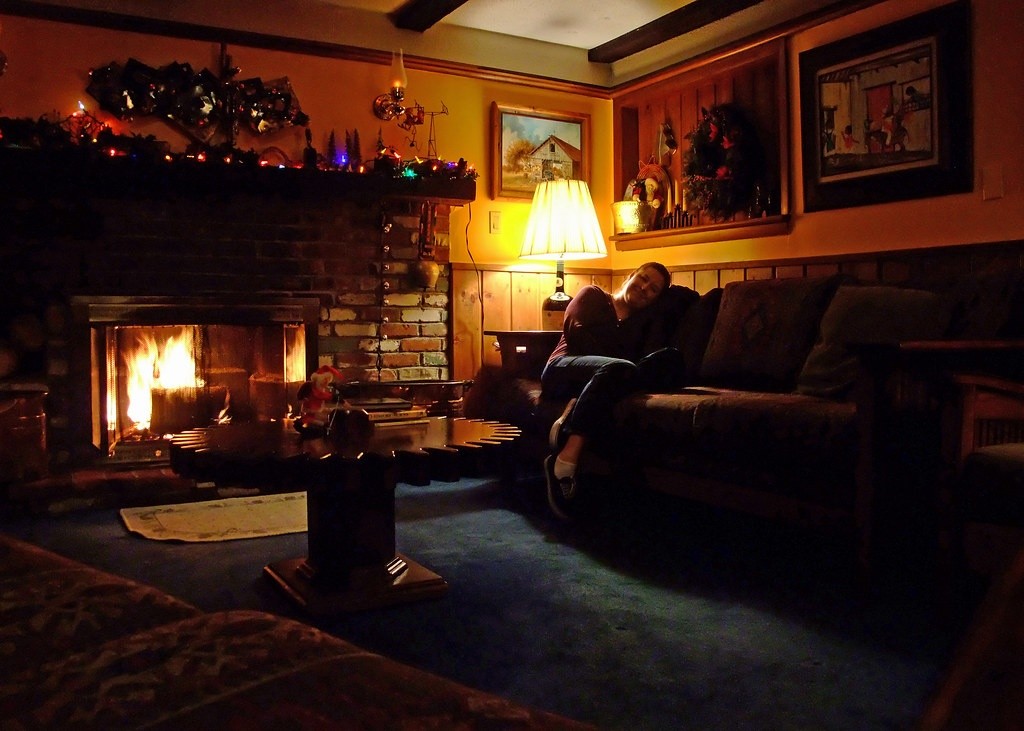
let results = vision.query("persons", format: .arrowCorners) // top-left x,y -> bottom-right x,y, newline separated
632,174 -> 666,208
542,261 -> 684,522
299,364 -> 343,427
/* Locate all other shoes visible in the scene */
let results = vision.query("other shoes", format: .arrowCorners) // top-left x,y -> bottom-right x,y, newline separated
544,455 -> 576,519
549,397 -> 577,449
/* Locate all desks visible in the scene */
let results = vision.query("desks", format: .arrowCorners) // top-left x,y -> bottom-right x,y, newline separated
168,413 -> 523,618
484,329 -> 565,371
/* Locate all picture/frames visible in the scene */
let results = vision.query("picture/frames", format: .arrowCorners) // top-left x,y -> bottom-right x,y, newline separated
491,100 -> 591,201
798,0 -> 973,212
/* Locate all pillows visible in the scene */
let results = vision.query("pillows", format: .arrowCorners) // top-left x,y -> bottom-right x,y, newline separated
698,276 -> 836,389
795,281 -> 961,397
640,285 -> 722,385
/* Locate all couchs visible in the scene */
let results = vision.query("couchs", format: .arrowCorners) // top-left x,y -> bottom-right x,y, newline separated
498,276 -> 1024,731
0,530 -> 602,731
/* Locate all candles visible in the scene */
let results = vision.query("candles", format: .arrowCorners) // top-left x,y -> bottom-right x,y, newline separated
674,179 -> 679,205
683,189 -> 688,212
667,184 -> 672,213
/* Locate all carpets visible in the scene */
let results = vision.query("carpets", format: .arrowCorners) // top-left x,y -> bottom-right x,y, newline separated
121,490 -> 307,544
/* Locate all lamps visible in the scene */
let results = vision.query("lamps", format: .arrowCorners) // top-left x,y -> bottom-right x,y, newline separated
520,180 -> 607,330
373,48 -> 409,121
